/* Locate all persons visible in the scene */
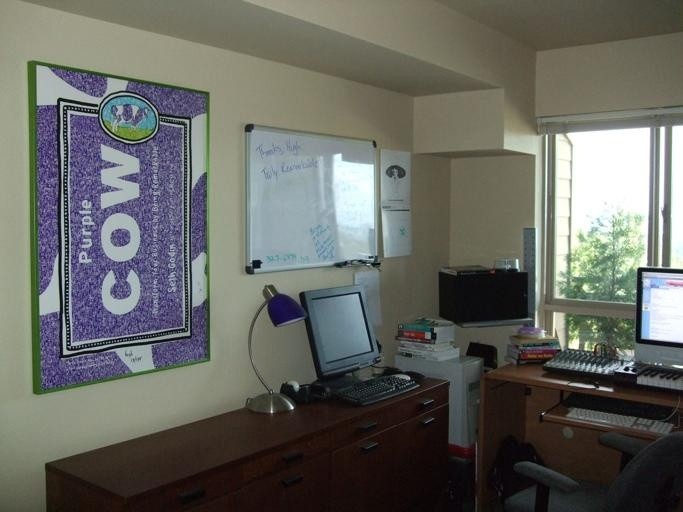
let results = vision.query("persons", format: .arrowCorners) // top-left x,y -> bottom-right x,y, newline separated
390,166 -> 404,200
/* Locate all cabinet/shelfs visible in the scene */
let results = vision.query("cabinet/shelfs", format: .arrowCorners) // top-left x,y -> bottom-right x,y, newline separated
46,379 -> 451,512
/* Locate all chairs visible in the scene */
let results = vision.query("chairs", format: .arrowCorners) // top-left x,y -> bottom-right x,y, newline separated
505,431 -> 683,512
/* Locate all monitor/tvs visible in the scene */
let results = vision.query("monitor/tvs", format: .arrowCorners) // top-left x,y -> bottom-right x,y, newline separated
633,266 -> 682,368
298,283 -> 381,403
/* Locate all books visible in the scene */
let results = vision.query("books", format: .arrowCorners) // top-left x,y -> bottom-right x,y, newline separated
394,317 -> 460,363
503,327 -> 562,366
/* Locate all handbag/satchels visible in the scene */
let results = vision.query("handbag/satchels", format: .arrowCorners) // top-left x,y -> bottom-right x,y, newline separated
487,437 -> 545,503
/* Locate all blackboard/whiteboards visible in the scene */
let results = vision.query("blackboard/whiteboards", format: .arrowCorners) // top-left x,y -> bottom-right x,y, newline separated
245,124 -> 379,274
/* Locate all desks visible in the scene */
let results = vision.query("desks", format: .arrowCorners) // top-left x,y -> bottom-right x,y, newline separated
478,357 -> 683,512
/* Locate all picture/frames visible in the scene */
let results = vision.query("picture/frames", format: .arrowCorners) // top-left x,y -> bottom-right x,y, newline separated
27,60 -> 211,395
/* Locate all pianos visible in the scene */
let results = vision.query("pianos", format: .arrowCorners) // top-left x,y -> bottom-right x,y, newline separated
613,360 -> 683,395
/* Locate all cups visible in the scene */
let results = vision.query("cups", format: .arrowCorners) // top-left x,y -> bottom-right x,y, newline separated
506,258 -> 519,269
496,259 -> 506,269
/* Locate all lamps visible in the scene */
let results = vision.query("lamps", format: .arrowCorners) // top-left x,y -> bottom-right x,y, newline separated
245,284 -> 308,415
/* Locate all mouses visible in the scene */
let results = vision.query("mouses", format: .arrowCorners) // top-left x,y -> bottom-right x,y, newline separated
391,373 -> 411,380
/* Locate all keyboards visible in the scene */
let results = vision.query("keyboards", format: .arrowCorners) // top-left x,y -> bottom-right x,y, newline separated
566,406 -> 674,438
331,375 -> 420,407
